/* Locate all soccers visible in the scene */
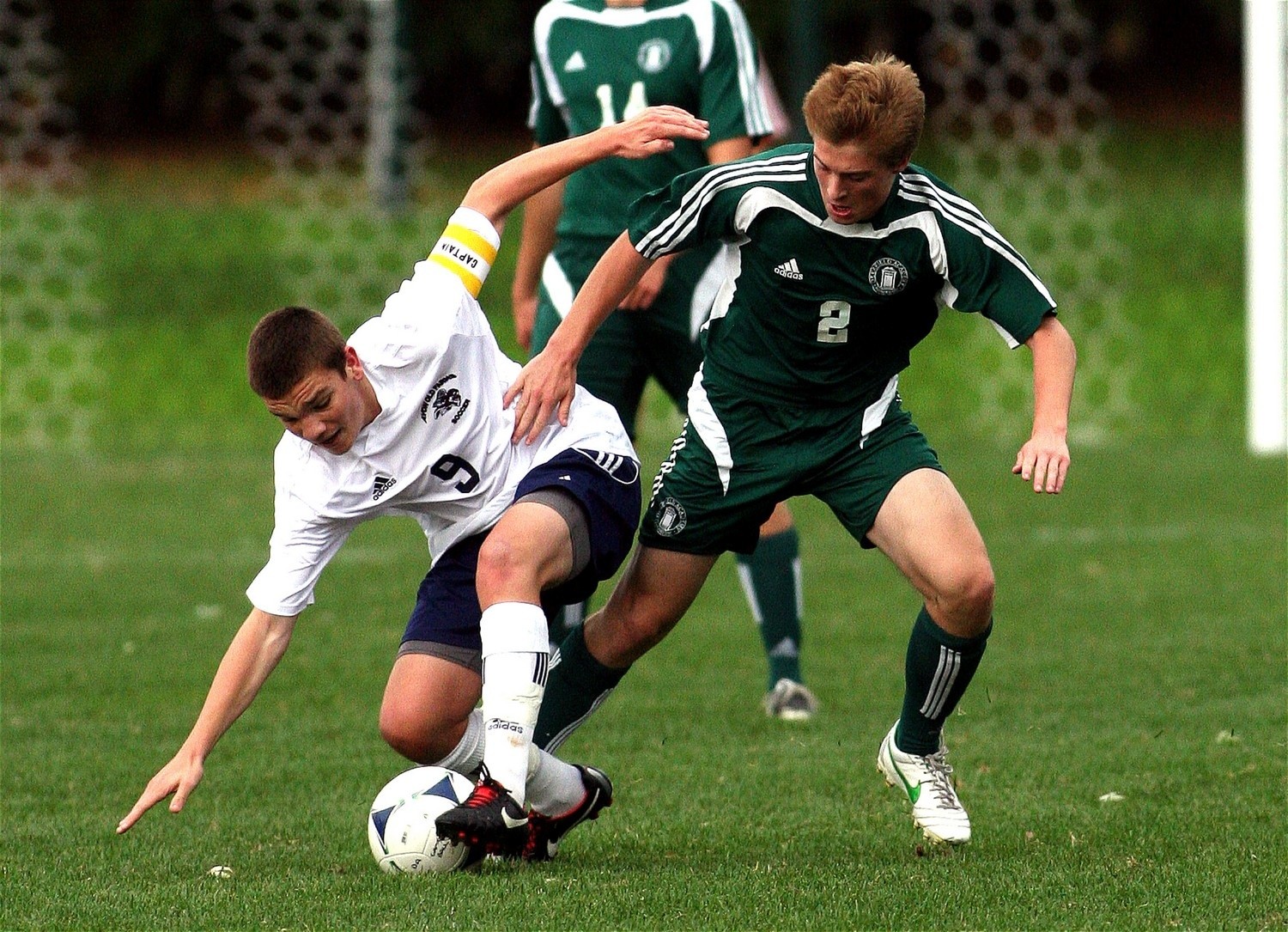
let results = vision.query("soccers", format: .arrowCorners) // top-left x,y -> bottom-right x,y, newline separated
368,767 -> 478,876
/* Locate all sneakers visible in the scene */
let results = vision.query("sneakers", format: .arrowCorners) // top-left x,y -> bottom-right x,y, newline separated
436,762 -> 529,860
765,679 -> 815,722
522,763 -> 613,862
877,719 -> 970,847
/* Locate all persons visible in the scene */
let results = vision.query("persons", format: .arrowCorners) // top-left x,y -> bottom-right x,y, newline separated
110,103 -> 710,859
535,54 -> 1075,844
510,0 -> 815,722
